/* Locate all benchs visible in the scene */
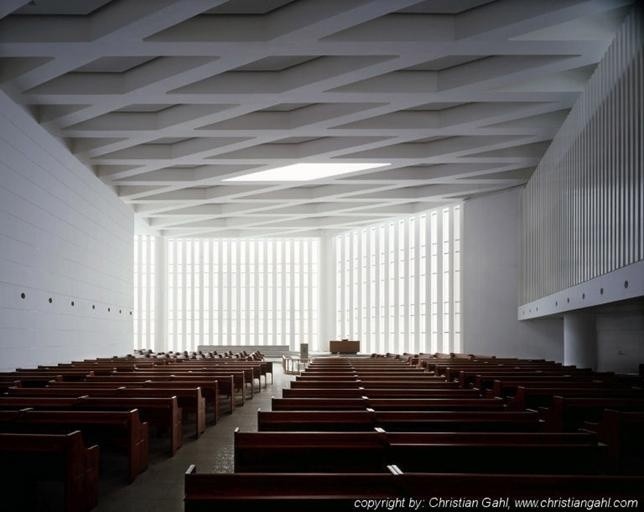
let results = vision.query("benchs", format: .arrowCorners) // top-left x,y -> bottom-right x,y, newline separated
283,388 -> 481,398
234,432 -> 598,472
16,352 -> 277,389
184,473 -> 644,512
0,430 -> 99,509
296,357 -> 446,380
0,408 -> 148,485
538,395 -> 644,445
259,411 -> 540,432
0,380 -> 221,425
291,381 -> 461,389
272,398 -> 514,410
0,396 -> 183,457
485,379 -> 621,397
0,375 -> 236,414
446,357 -> 616,378
582,408 -> 644,473
0,386 -> 206,440
507,386 -> 644,410
